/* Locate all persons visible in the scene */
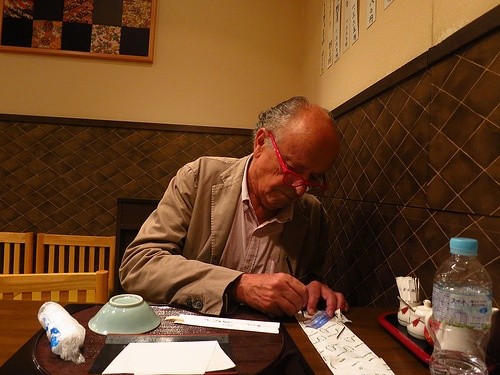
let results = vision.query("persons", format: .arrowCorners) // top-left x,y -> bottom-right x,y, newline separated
119,96 -> 350,318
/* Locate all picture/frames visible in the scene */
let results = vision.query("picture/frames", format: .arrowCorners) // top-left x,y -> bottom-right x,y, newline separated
0,0 -> 157,64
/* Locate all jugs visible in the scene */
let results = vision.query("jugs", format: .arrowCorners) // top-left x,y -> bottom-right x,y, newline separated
425,308 -> 499,356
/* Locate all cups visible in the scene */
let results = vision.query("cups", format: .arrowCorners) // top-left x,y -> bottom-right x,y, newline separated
407,300 -> 433,341
397,300 -> 422,326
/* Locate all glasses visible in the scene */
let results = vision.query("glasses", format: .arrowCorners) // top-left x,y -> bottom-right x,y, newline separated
266,130 -> 327,194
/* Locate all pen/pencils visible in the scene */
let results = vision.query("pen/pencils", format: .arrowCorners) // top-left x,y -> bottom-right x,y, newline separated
285,255 -> 305,318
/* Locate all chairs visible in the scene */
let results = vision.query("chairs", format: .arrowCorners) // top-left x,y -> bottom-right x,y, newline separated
0,232 -> 116,303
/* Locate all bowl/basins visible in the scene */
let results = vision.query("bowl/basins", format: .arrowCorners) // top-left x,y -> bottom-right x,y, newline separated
87,293 -> 161,336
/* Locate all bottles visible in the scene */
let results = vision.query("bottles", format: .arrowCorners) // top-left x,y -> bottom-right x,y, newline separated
429,237 -> 493,375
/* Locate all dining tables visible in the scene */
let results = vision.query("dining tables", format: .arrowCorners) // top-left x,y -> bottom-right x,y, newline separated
0,299 -> 430,375
